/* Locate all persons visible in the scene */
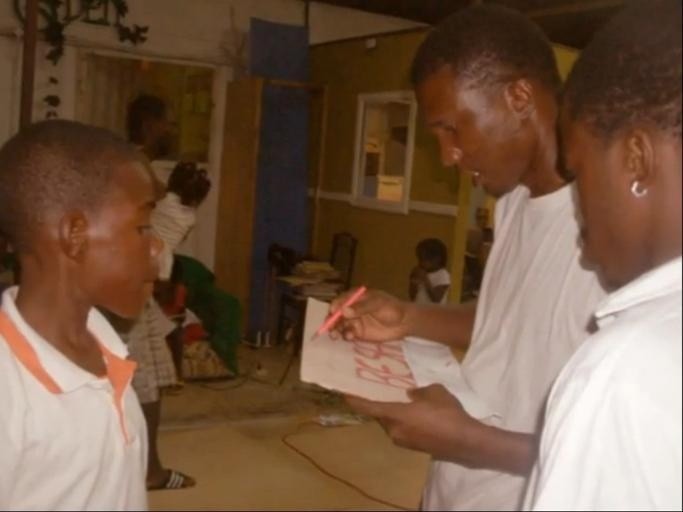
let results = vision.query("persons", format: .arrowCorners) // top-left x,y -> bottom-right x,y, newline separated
108,303 -> 198,489
144,160 -> 212,288
0,120 -> 164,511
324,6 -> 612,511
411,236 -> 452,306
522,2 -> 683,511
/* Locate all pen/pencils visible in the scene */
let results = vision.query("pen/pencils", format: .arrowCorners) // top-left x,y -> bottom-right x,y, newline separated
309,287 -> 365,344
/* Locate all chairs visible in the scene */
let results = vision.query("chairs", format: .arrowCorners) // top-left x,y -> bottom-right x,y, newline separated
158,245 -> 241,392
270,230 -> 356,350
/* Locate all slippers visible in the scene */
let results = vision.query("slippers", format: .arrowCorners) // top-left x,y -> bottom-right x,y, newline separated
147,469 -> 197,488
